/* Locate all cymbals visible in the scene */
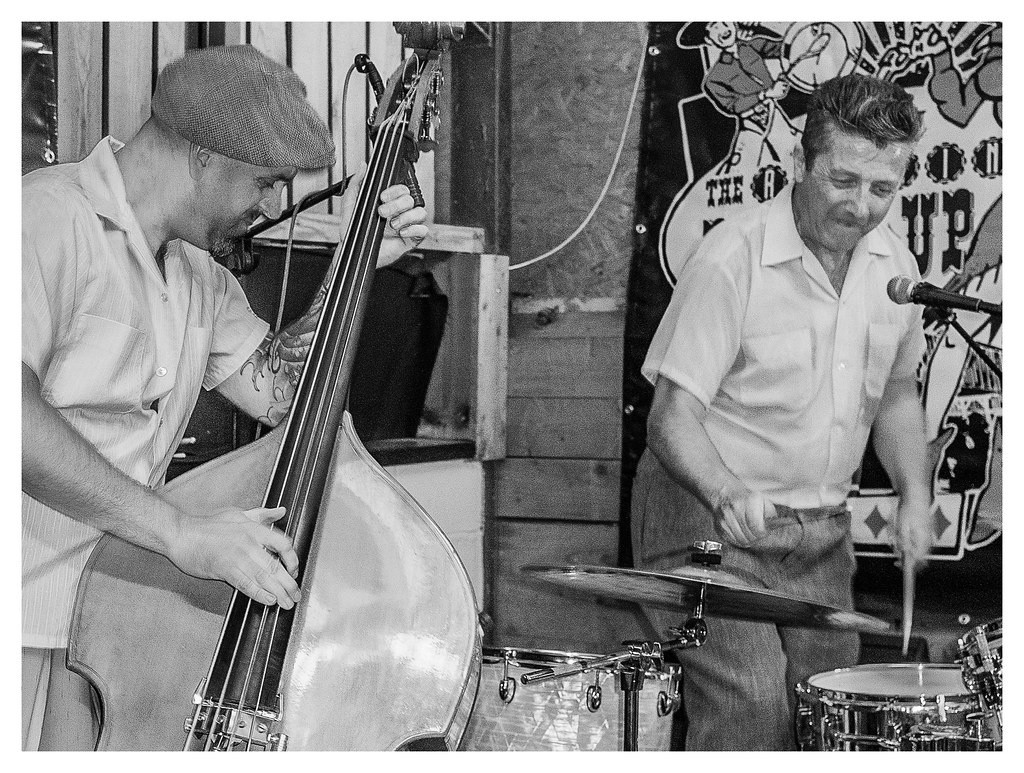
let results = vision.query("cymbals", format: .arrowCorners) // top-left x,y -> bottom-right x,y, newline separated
522,559 -> 892,635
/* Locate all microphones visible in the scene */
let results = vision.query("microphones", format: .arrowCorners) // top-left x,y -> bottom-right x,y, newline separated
888,276 -> 1003,315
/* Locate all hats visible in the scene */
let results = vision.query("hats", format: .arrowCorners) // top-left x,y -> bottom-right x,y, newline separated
150,43 -> 336,170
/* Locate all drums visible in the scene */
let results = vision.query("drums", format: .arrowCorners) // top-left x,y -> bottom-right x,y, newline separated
960,613 -> 1003,744
791,661 -> 980,753
461,641 -> 683,752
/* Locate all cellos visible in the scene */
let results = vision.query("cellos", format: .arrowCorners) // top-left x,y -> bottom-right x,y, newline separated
62,24 -> 487,755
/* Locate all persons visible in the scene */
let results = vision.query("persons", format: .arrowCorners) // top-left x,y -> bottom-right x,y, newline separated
632,73 -> 931,750
24,42 -> 430,752
